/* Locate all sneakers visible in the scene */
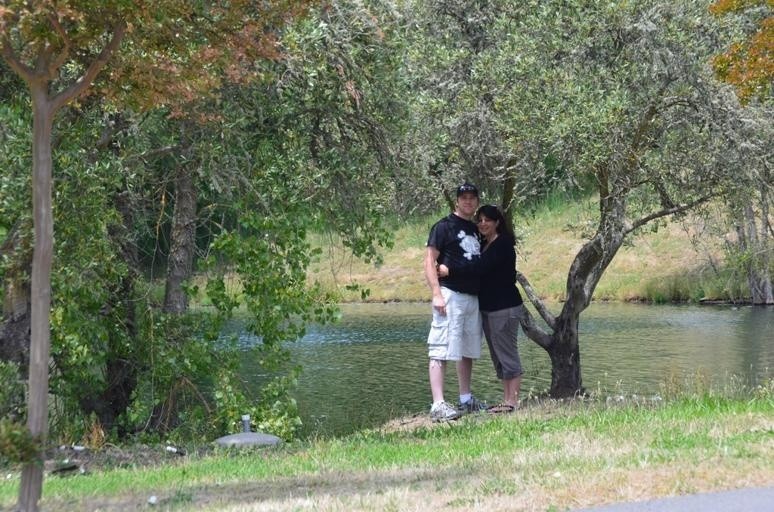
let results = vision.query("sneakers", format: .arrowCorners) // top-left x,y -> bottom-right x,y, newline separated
430,402 -> 462,421
457,396 -> 491,410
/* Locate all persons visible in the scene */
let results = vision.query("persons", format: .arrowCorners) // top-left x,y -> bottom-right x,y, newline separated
422,181 -> 493,422
435,202 -> 524,413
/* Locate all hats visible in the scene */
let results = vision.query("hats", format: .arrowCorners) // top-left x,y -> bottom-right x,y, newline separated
457,185 -> 476,196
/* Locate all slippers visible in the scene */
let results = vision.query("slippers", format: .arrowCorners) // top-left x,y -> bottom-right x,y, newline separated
486,404 -> 519,412
